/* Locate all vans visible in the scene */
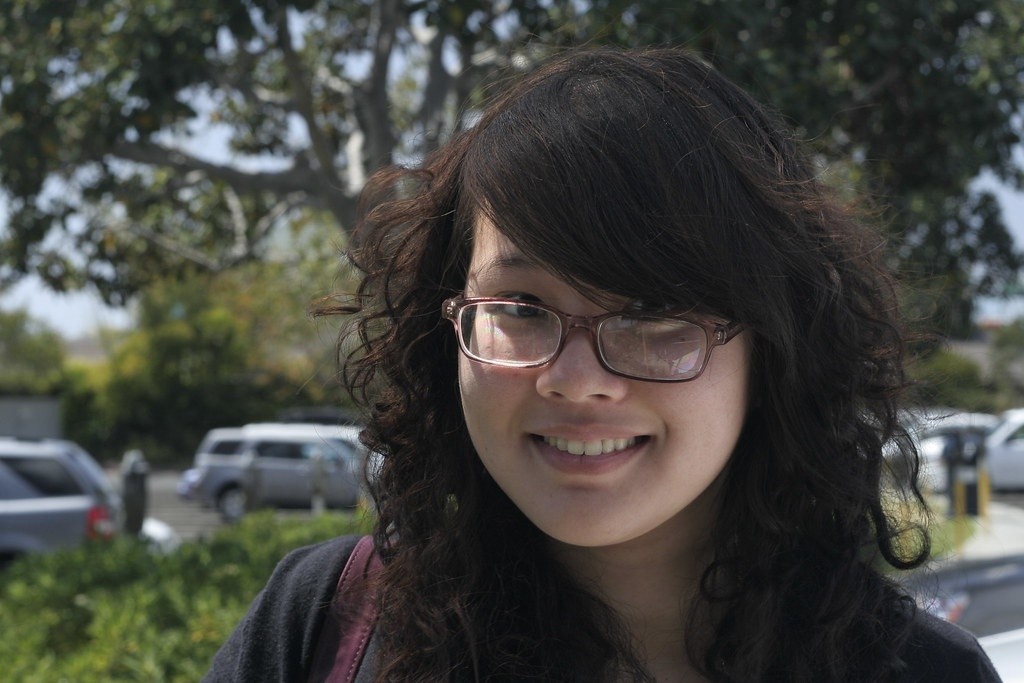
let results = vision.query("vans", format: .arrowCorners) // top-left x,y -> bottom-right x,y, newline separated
173,424 -> 375,523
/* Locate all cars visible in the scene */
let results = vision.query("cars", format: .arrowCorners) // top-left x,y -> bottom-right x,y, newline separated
0,433 -> 123,573
860,407 -> 1024,494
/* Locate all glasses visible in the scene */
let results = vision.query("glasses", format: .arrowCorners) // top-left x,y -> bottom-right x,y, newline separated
441,292 -> 751,383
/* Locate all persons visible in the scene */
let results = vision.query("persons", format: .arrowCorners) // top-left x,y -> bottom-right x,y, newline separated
201,45 -> 1002,683
120,449 -> 147,534
